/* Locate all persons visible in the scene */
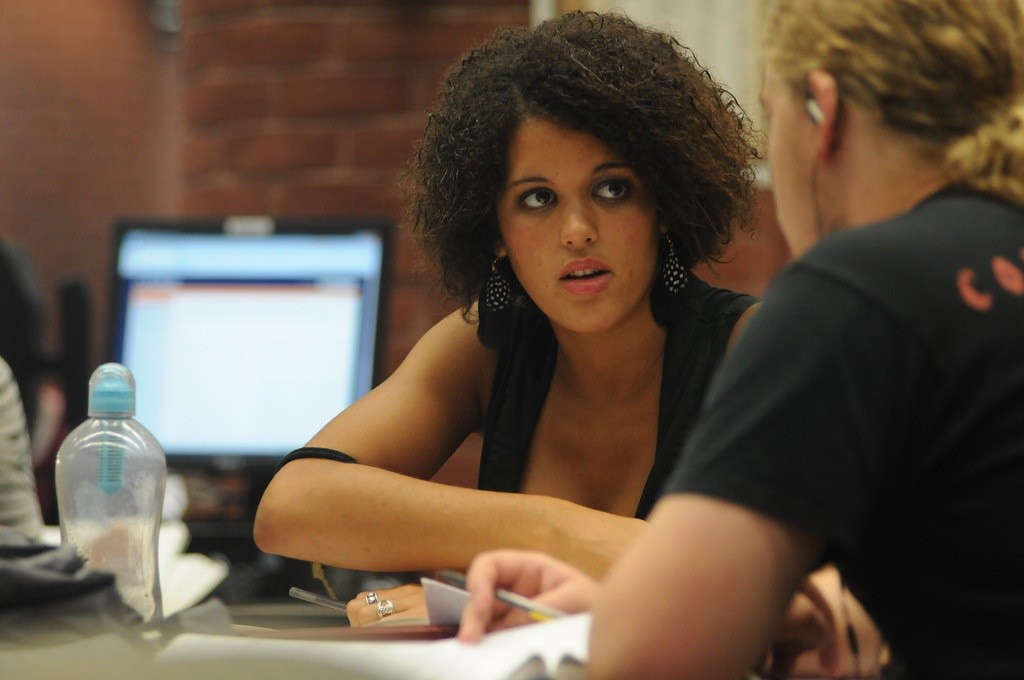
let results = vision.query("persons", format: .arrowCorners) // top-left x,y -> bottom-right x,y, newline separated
462,0 -> 1024,680
253,13 -> 771,636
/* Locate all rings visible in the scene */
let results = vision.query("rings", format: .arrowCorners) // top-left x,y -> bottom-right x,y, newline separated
365,592 -> 378,605
378,600 -> 394,619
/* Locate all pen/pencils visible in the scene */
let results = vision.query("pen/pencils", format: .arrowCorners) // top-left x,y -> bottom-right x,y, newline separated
289,586 -> 347,611
441,569 -> 566,619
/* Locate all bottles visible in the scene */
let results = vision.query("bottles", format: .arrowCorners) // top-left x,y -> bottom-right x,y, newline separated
53,363 -> 167,631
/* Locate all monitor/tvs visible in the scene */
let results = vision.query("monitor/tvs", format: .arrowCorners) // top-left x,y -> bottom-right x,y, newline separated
104,217 -> 396,519
